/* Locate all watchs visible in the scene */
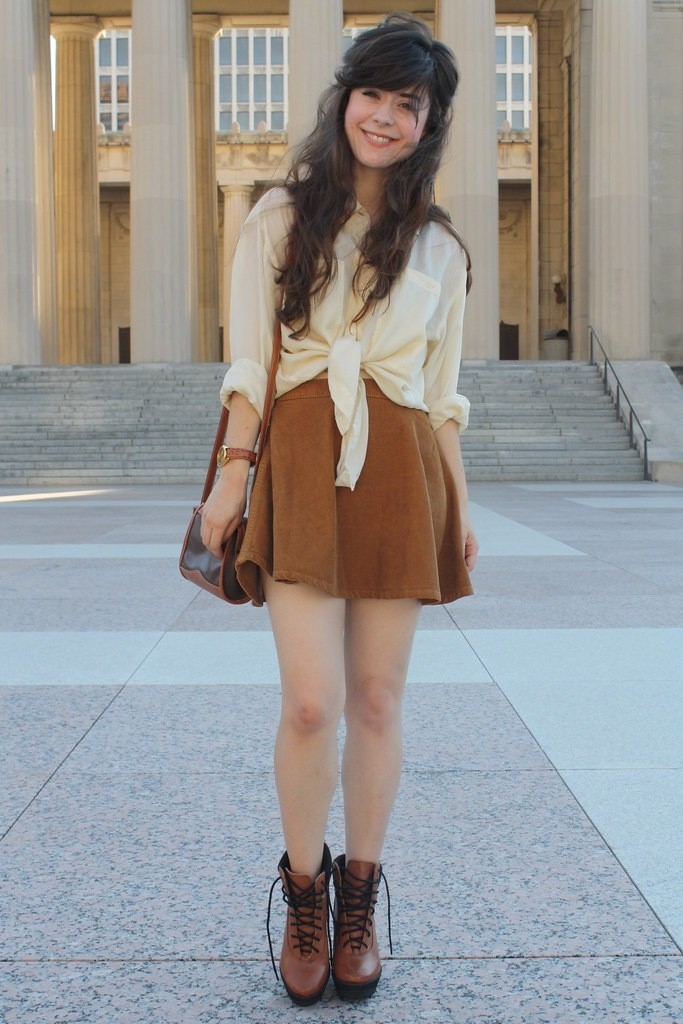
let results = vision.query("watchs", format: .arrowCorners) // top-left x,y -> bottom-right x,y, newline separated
216,444 -> 258,468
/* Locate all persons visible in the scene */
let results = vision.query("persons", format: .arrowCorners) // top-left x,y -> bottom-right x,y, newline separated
200,12 -> 479,1007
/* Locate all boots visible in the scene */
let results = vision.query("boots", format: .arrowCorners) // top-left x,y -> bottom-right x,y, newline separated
267,843 -> 393,1009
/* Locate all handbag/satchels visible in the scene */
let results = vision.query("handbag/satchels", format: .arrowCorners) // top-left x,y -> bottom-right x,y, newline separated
180,503 -> 258,605
